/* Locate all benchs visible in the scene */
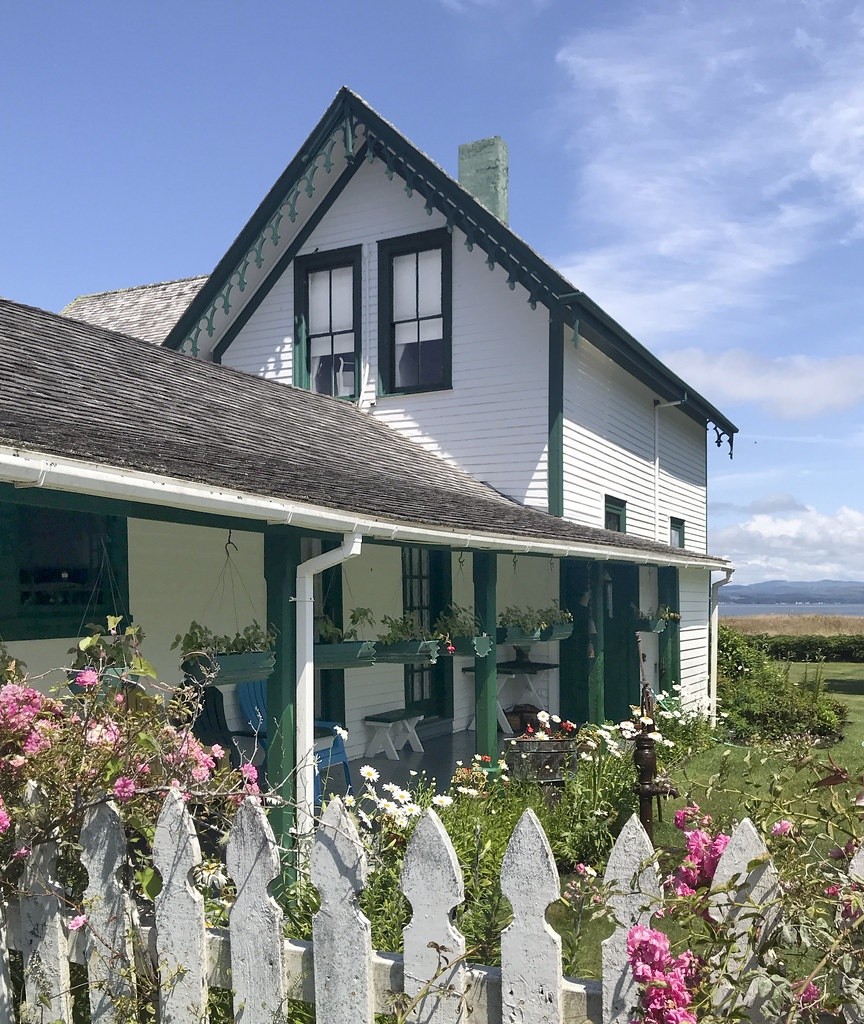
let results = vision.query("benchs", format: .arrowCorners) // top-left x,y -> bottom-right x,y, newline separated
362,708 -> 426,760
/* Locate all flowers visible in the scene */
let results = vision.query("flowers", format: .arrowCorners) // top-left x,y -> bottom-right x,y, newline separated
514,712 -> 579,739
379,610 -> 457,654
498,605 -> 576,633
638,605 -> 684,620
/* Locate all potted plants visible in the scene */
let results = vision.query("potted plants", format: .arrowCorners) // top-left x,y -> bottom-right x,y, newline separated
65,617 -> 158,696
171,617 -> 284,690
432,602 -> 493,657
312,606 -> 378,670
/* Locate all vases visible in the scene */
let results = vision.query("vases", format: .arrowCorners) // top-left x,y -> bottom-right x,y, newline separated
508,738 -> 577,785
544,622 -> 575,641
374,637 -> 440,666
631,616 -> 679,633
493,624 -> 542,644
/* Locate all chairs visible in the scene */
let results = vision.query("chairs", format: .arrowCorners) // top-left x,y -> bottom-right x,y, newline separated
231,678 -> 352,806
172,685 -> 272,807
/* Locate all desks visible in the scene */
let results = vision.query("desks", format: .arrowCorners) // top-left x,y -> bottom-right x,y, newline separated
461,663 -> 558,735
